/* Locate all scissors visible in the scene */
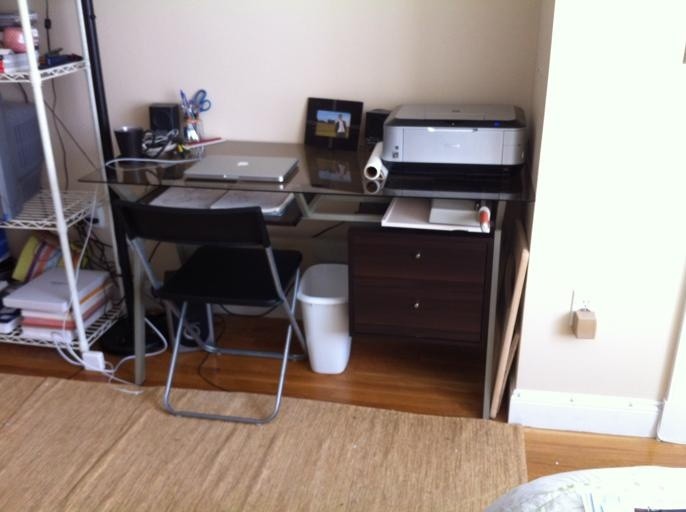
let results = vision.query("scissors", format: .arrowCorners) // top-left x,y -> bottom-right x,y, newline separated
192,90 -> 211,111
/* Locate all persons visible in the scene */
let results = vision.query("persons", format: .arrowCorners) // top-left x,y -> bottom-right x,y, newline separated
333,114 -> 346,138
334,161 -> 347,180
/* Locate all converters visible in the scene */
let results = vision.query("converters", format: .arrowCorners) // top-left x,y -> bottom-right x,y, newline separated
82,350 -> 106,372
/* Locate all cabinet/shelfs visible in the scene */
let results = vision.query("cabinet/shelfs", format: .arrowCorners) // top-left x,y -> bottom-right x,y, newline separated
349,228 -> 509,360
0,0 -> 134,354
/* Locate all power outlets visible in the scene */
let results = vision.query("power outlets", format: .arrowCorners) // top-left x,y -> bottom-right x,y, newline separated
569,293 -> 600,334
84,206 -> 105,228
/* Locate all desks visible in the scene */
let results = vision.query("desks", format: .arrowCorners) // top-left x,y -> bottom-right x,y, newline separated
79,141 -> 524,420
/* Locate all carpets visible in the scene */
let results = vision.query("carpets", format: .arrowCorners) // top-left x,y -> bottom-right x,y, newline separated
0,370 -> 527,512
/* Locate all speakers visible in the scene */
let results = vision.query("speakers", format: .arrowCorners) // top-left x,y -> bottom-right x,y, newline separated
161,269 -> 215,354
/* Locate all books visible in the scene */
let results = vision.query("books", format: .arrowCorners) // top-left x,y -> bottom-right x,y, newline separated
428,200 -> 485,228
0,269 -> 115,344
0,10 -> 41,78
148,183 -> 226,210
212,189 -> 296,215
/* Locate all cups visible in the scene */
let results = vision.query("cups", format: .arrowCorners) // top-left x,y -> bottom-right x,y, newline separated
114,126 -> 155,155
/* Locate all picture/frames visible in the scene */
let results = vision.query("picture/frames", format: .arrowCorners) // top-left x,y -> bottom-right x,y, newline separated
303,98 -> 363,149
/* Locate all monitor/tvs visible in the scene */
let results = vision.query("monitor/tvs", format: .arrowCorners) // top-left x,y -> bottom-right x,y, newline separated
0,101 -> 45,222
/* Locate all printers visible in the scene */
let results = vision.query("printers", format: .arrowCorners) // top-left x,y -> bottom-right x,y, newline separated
381,102 -> 528,202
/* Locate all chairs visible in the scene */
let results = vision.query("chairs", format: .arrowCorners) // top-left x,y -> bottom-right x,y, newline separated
111,198 -> 309,423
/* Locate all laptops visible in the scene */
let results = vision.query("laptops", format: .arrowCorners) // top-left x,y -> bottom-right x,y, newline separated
183,154 -> 298,183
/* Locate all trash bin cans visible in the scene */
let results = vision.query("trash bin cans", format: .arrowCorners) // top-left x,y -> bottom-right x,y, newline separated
297,263 -> 352,375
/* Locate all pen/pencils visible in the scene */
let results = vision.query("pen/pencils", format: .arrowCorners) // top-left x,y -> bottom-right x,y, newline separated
178,89 -> 201,142
